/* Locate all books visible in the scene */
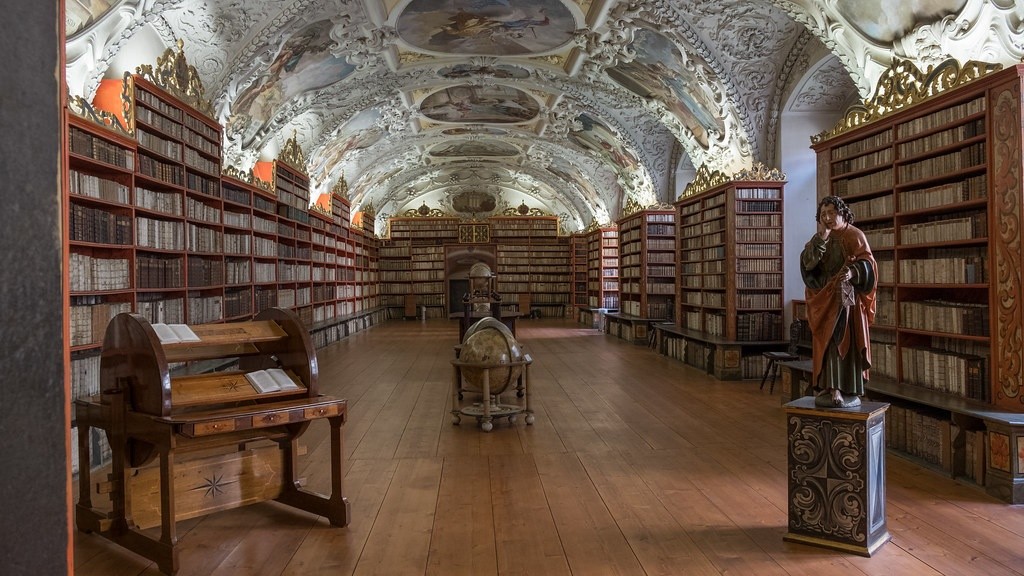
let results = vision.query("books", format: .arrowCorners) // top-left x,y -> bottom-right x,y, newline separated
829,97 -> 991,488
243,369 -> 299,394
67,82 -> 381,476
383,240 -> 571,320
151,324 -> 202,345
571,187 -> 782,378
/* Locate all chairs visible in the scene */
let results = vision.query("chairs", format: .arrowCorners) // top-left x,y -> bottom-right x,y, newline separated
760,320 -> 806,394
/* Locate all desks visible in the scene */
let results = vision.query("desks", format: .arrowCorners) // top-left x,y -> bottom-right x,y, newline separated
781,396 -> 892,559
449,311 -> 525,344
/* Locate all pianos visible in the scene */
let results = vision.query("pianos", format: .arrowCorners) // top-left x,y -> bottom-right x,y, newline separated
73,305 -> 350,576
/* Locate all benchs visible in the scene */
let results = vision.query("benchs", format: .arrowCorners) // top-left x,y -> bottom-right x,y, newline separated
653,325 -> 743,382
307,305 -> 388,353
781,358 -> 1024,505
577,306 -> 608,328
69,357 -> 240,426
605,313 -> 649,345
390,304 -> 445,320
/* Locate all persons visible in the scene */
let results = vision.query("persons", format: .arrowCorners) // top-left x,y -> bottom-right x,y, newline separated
800,197 -> 879,406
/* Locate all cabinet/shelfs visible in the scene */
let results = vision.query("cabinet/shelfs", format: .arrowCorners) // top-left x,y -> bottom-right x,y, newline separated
317,169 -> 351,229
499,237 -> 571,319
335,225 -> 355,318
606,197 -> 677,344
278,201 -> 313,328
575,217 -> 619,328
250,128 -> 311,211
487,196 -> 558,236
351,197 -> 376,238
356,221 -> 369,311
370,234 -> 381,308
388,199 -> 459,237
67,117 -> 139,395
565,229 -> 587,317
648,161 -> 787,383
223,162 -> 279,324
309,203 -> 336,322
95,35 -> 225,174
808,54 -> 1024,506
132,145 -> 223,328
381,237 -> 445,321
